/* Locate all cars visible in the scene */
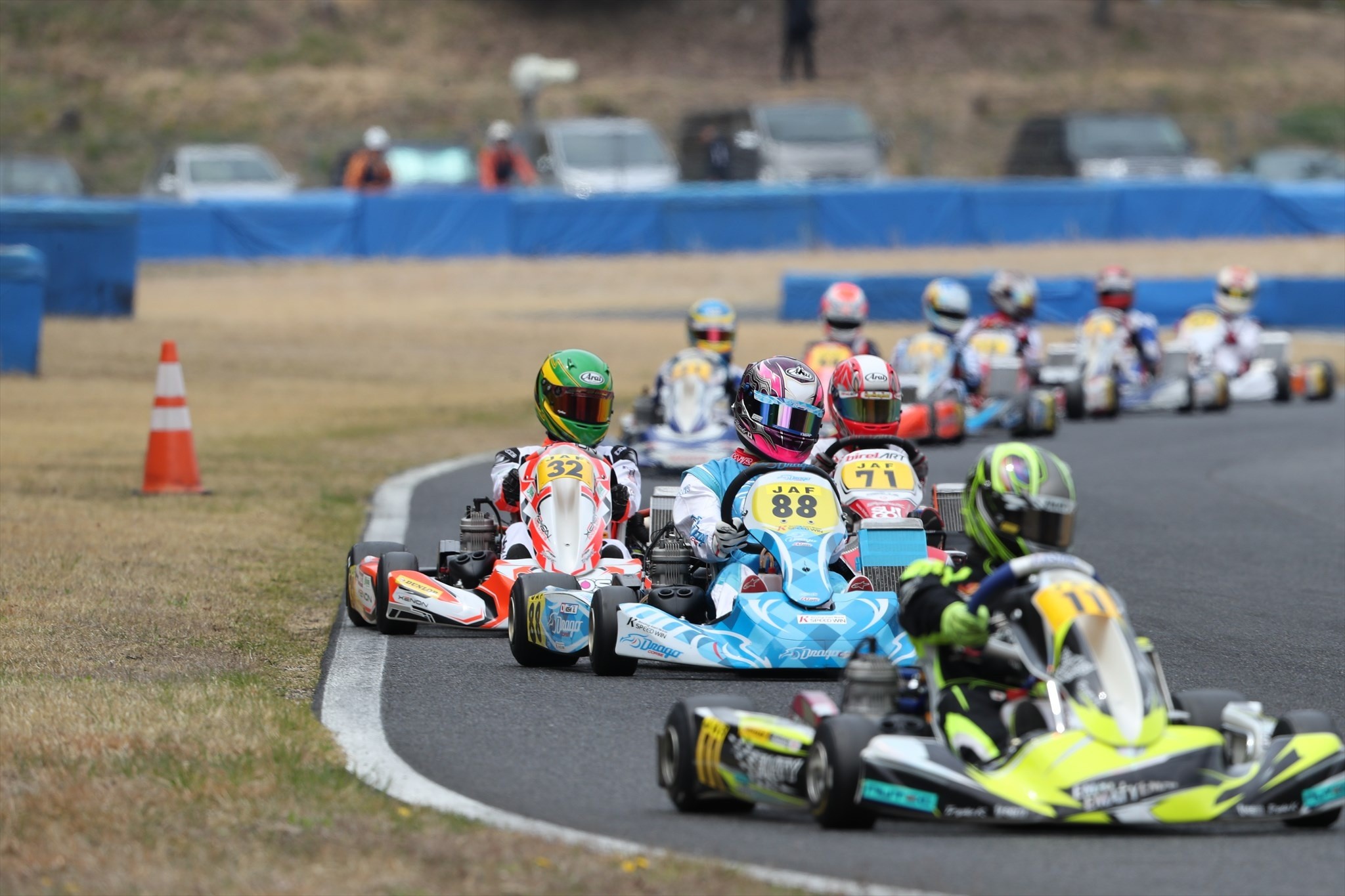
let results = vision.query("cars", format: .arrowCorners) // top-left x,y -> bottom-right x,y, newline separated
530,118 -> 684,199
0,154 -> 90,199
1240,143 -> 1345,182
155,143 -> 301,199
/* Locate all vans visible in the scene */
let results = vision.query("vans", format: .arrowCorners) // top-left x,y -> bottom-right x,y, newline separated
678,98 -> 880,189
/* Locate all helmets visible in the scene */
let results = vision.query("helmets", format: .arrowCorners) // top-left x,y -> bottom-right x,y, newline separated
822,282 -> 868,344
987,269 -> 1040,318
828,354 -> 902,448
960,442 -> 1077,563
1095,266 -> 1136,311
732,356 -> 825,463
688,296 -> 736,363
535,349 -> 614,449
1214,267 -> 1259,313
921,278 -> 970,333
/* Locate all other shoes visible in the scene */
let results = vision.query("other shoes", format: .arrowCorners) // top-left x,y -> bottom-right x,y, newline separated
921,507 -> 945,547
1005,700 -> 1048,742
503,543 -> 532,560
844,575 -> 874,592
741,575 -> 769,593
601,544 -> 627,558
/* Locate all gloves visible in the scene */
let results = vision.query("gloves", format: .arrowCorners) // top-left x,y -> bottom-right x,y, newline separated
612,483 -> 630,521
940,600 -> 990,649
811,453 -> 837,474
841,505 -> 854,532
503,468 -> 520,506
714,517 -> 749,554
909,449 -> 929,479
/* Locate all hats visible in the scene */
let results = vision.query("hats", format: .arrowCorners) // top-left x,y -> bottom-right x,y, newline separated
487,121 -> 511,141
363,125 -> 391,152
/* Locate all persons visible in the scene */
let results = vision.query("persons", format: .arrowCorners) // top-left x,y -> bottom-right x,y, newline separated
480,120 -> 537,190
672,357 -> 874,622
698,120 -> 734,179
896,442 -> 1185,771
491,351 -> 641,559
648,258 -> 1259,424
813,355 -> 946,549
343,127 -> 391,192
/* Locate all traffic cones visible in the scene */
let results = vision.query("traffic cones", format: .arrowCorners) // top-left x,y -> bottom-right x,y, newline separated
124,338 -> 215,497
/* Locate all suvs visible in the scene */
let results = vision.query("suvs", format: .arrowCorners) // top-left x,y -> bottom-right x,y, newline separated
1000,109 -> 1218,183
